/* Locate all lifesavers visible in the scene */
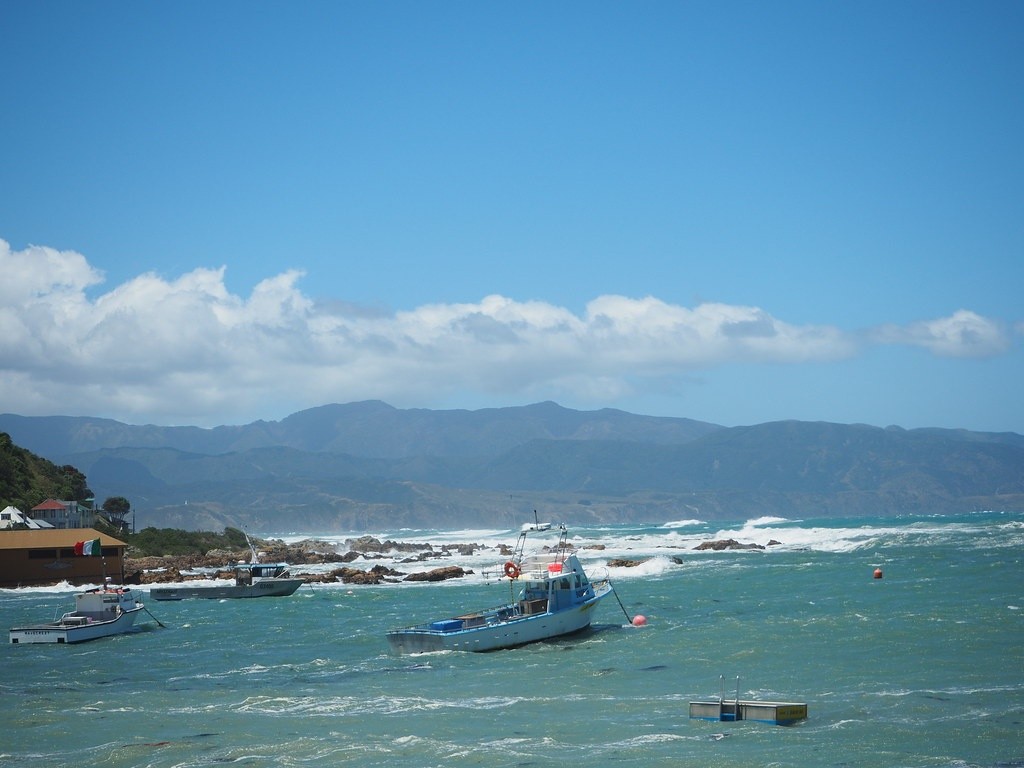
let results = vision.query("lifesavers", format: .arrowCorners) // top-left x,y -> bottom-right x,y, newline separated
498,611 -> 508,620
549,568 -> 561,571
505,563 -> 519,577
549,564 -> 563,568
116,605 -> 120,613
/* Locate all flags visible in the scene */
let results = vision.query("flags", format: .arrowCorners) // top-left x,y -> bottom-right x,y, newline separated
74,537 -> 101,556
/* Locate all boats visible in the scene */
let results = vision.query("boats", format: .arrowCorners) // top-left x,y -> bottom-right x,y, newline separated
148,562 -> 309,601
528,522 -> 551,532
9,537 -> 148,645
384,511 -> 613,655
